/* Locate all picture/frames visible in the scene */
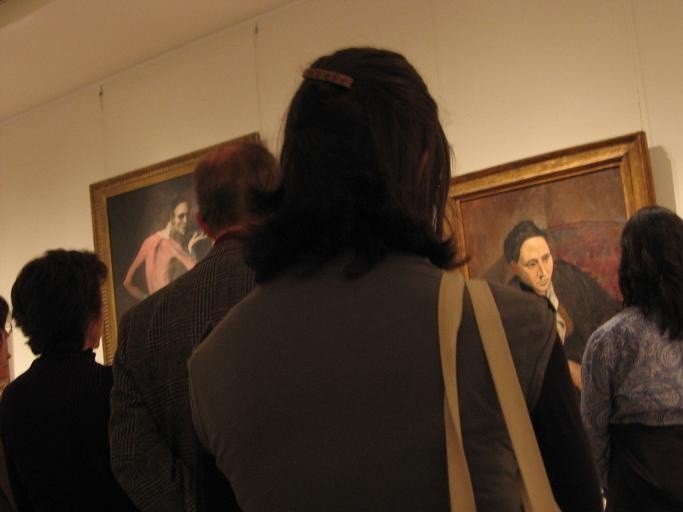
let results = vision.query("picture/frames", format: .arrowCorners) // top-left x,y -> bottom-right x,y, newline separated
439,131 -> 657,399
85,131 -> 262,366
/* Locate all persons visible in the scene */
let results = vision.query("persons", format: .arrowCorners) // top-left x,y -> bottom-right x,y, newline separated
0,248 -> 143,512
108,141 -> 281,512
580,205 -> 683,512
188,47 -> 603,512
0,296 -> 12,380
123,196 -> 207,301
504,219 -> 624,394
